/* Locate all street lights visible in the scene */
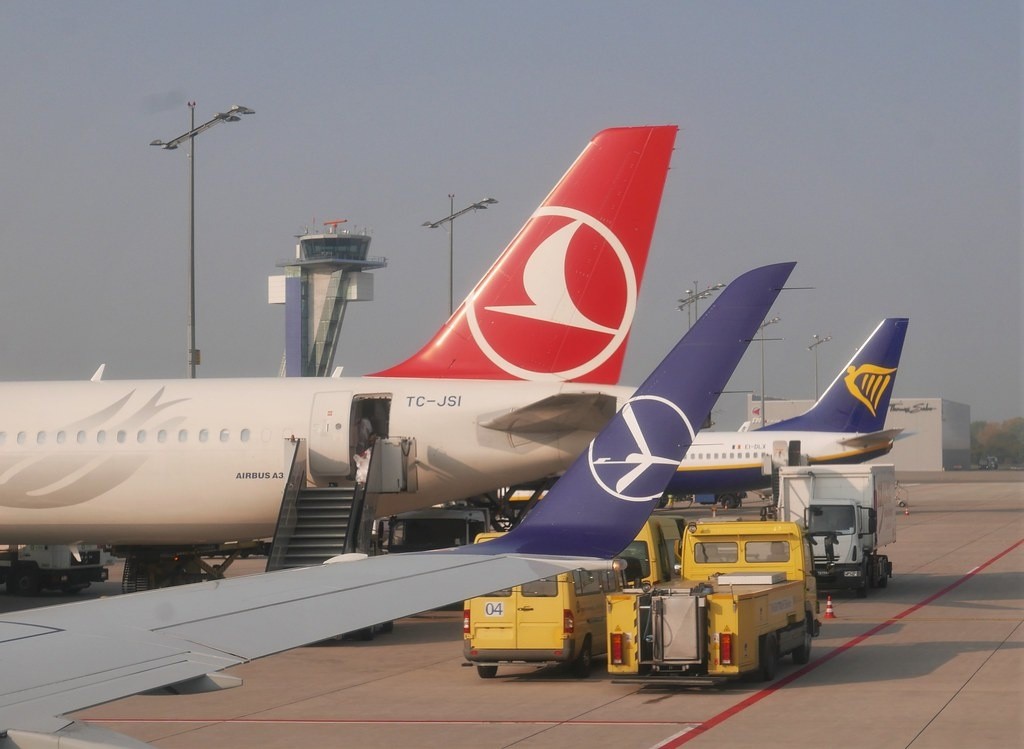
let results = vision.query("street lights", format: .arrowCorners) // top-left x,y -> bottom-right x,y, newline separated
807,334 -> 832,402
148,99 -> 255,379
674,281 -> 728,329
757,315 -> 782,428
418,193 -> 501,317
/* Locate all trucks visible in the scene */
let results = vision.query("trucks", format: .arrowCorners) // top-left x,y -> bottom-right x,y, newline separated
374,507 -> 490,610
760,463 -> 898,598
604,520 -> 835,687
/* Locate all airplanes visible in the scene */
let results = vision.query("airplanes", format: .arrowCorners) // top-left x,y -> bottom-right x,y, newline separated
0,125 -> 922,748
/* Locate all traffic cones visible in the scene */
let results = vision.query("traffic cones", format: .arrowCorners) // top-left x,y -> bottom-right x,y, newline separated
903,507 -> 909,515
822,594 -> 837,619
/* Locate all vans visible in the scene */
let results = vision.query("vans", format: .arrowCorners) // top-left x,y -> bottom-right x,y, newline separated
460,531 -> 643,678
619,514 -> 689,591
